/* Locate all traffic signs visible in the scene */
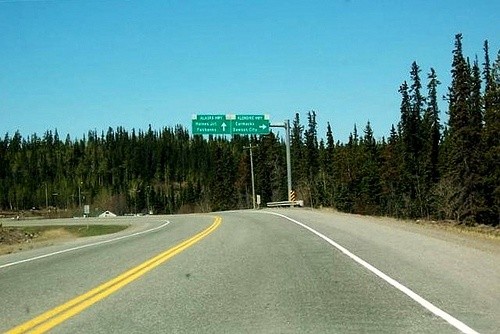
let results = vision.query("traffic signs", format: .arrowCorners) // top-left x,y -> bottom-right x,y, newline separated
192,115 -> 270,135
